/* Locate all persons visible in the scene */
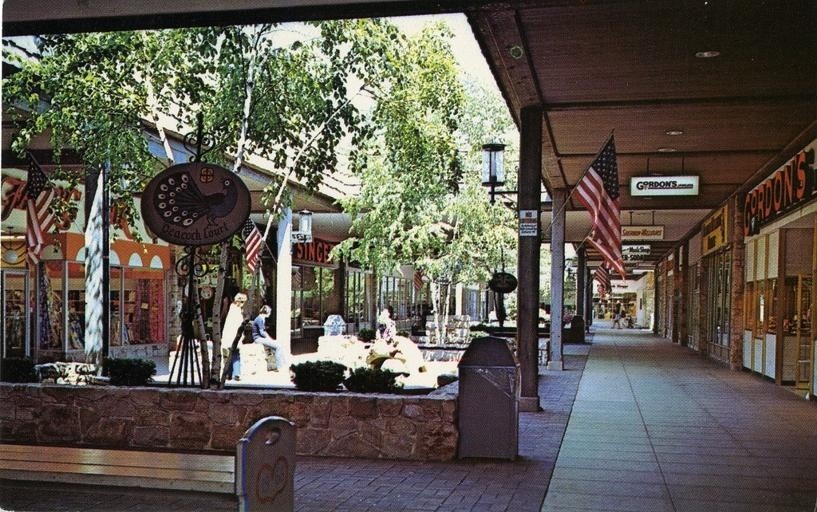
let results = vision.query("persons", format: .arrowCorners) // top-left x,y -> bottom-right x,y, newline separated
612,300 -> 637,327
220,291 -> 248,381
250,305 -> 281,372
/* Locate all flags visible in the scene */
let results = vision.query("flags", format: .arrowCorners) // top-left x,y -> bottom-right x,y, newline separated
574,133 -> 626,295
413,267 -> 423,293
25,155 -> 61,265
244,216 -> 262,273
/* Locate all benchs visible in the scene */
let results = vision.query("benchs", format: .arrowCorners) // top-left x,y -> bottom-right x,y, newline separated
0,415 -> 297,512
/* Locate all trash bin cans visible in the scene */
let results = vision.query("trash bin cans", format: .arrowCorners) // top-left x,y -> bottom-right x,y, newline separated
457,337 -> 521,460
571,315 -> 585,343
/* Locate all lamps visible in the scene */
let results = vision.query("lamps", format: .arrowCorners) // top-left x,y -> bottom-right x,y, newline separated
5,227 -> 18,263
565,258 -> 578,281
481,140 -> 518,205
289,208 -> 312,245
